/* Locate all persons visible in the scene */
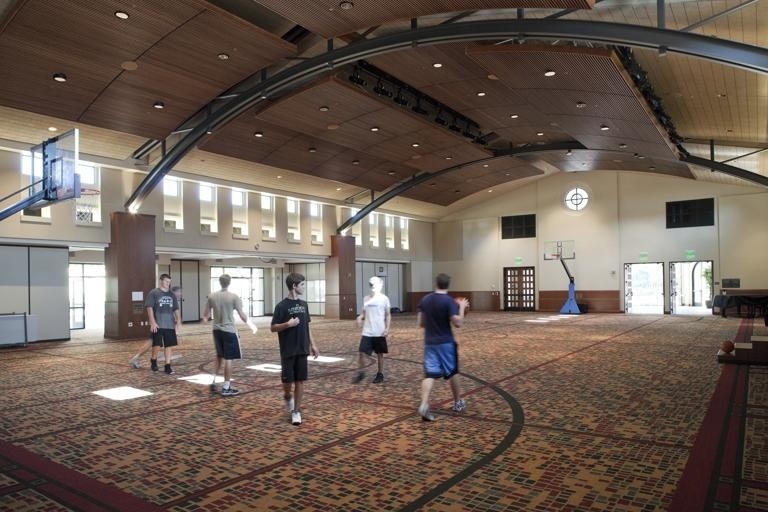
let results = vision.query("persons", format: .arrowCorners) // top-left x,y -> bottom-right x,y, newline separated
130,285 -> 182,368
199,273 -> 257,396
418,272 -> 470,422
269,273 -> 319,425
352,276 -> 392,383
143,273 -> 182,375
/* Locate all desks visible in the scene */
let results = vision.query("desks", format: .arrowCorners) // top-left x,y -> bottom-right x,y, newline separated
708,294 -> 767,327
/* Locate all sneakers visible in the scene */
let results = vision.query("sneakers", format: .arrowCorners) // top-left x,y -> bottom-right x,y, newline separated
418,404 -> 435,421
452,399 -> 466,412
373,372 -> 385,383
221,386 -> 240,397
128,356 -> 144,368
350,371 -> 367,384
165,364 -> 173,374
283,393 -> 294,413
291,410 -> 302,425
208,383 -> 218,395
150,358 -> 158,371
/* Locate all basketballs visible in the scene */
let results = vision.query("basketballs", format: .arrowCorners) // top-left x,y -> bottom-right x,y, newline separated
454,297 -> 469,317
720,340 -> 734,352
